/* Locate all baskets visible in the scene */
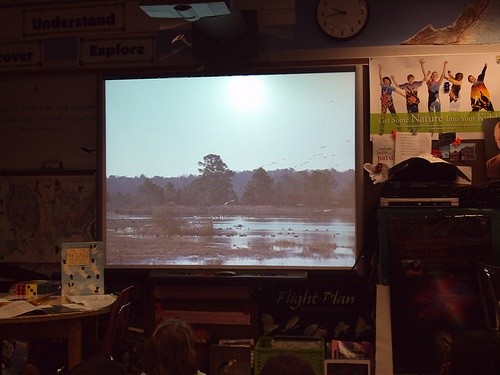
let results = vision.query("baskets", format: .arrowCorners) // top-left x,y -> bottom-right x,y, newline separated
255,335 -> 325,375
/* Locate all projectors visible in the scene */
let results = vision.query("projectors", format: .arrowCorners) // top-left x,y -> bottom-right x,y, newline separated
138,0 -> 231,22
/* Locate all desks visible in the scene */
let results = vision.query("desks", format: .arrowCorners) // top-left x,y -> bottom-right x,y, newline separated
378,207 -> 500,375
156,299 -> 262,375
0,293 -> 118,371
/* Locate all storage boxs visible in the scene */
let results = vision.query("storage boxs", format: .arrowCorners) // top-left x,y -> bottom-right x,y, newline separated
209,332 -> 324,375
16,280 -> 50,295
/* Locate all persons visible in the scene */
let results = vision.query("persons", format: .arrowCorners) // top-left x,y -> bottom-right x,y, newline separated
443,71 -> 464,112
390,71 -> 431,136
468,63 -> 495,112
418,58 -> 448,134
377,64 -> 406,136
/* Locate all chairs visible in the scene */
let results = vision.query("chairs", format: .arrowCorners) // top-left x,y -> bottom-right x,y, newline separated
68,291 -> 132,375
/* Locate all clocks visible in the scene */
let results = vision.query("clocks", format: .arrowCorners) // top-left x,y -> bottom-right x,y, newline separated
315,0 -> 369,41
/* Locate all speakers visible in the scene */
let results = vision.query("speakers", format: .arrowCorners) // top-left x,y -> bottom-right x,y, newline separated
209,346 -> 251,375
